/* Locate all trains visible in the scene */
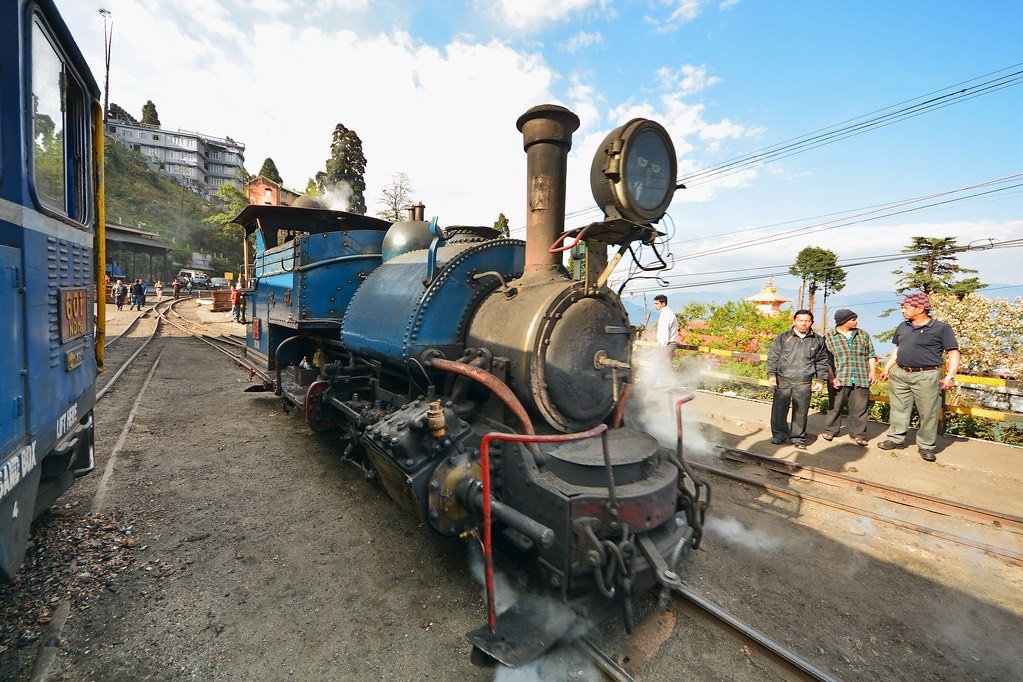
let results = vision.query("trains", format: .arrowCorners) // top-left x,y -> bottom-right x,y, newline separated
225,103 -> 714,673
0,0 -> 108,596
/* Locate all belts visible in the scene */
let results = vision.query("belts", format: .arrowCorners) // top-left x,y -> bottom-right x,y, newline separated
898,364 -> 937,372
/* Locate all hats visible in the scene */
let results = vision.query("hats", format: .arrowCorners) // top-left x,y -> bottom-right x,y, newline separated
901,293 -> 931,310
834,309 -> 858,325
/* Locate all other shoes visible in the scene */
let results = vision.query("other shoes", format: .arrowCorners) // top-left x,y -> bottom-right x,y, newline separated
794,442 -> 806,449
822,432 -> 833,441
771,437 -> 783,444
138,310 -> 141,311
877,439 -> 904,450
234,319 -> 239,323
118,309 -> 119,310
850,436 -> 868,446
130,308 -> 132,310
120,308 -> 122,311
918,448 -> 936,460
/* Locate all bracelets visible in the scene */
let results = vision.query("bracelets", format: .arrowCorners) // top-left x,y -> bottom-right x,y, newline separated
946,375 -> 955,380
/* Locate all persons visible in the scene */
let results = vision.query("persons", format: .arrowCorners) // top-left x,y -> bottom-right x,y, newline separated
822,309 -> 878,446
236,278 -> 243,298
111,275 -> 192,311
767,309 -> 829,449
230,284 -> 240,323
205,277 -> 211,290
651,294 -> 679,392
878,294 -> 961,462
